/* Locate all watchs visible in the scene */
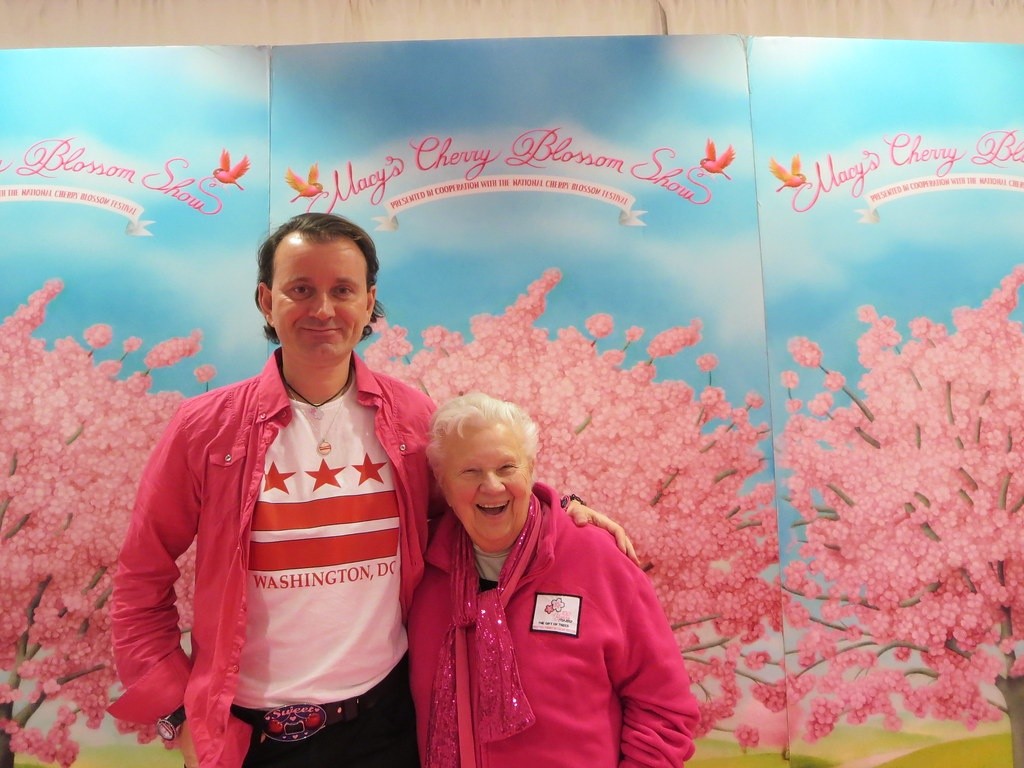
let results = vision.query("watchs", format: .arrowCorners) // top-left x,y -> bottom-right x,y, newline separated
156,706 -> 186,742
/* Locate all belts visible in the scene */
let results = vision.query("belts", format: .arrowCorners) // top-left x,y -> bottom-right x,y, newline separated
231,670 -> 407,742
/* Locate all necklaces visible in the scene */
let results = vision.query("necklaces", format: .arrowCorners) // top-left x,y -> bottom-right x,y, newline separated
281,363 -> 352,455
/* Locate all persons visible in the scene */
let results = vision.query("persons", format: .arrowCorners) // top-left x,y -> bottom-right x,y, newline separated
407,390 -> 701,768
110,212 -> 642,768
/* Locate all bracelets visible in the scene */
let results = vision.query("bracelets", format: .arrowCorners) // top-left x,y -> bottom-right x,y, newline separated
561,495 -> 586,511
